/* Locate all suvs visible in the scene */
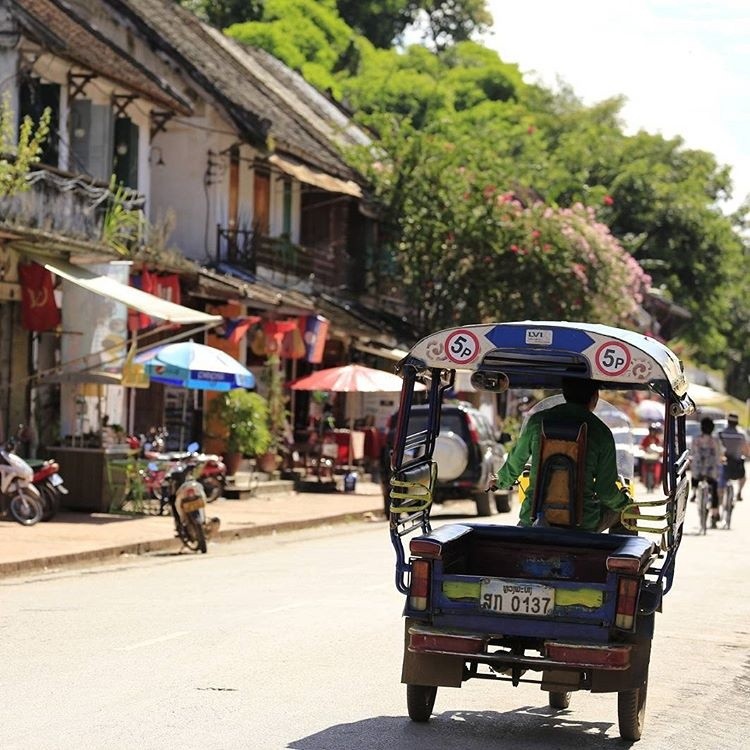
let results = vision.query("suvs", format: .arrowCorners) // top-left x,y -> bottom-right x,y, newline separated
384,405 -> 518,516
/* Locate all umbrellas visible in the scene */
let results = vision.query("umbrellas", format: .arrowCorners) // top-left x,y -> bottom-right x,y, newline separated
283,362 -> 429,476
128,341 -> 256,456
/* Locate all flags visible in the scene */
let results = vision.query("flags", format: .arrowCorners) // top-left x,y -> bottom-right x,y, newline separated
118,262 -> 330,365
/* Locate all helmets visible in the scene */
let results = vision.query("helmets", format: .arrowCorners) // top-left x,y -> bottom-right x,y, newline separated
649,423 -> 657,433
656,421 -> 664,432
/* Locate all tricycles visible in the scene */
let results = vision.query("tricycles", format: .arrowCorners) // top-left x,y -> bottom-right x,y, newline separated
379,319 -> 699,741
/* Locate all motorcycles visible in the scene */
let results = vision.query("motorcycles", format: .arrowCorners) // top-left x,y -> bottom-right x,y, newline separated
146,455 -> 227,504
0,422 -> 73,527
147,442 -> 212,556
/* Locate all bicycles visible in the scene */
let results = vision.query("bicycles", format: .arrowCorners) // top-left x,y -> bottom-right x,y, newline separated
696,475 -> 737,532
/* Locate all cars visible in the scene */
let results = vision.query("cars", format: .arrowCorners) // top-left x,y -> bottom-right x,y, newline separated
612,421 -> 740,474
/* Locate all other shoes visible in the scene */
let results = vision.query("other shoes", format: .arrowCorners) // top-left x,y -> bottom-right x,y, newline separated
637,469 -> 745,531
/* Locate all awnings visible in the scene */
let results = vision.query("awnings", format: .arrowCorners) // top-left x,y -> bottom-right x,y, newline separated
4,241 -> 226,388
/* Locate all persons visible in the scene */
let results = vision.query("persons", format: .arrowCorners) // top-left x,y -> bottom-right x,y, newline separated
688,416 -> 727,522
638,422 -> 664,485
485,381 -> 641,536
717,412 -> 750,511
